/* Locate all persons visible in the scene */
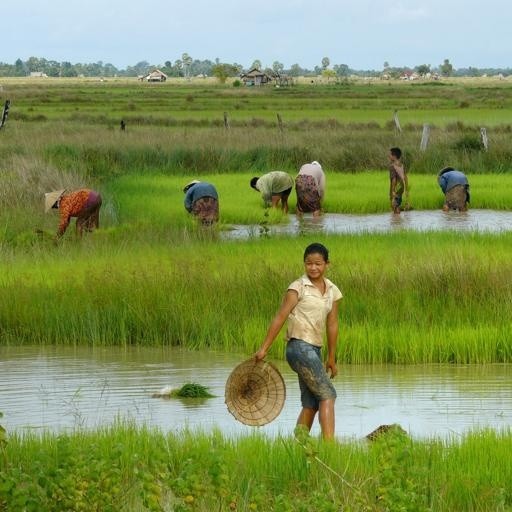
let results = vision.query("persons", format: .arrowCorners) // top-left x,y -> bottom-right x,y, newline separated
250,171 -> 294,214
183,180 -> 219,228
388,148 -> 408,214
295,161 -> 324,219
438,166 -> 470,212
255,243 -> 343,438
45,189 -> 102,240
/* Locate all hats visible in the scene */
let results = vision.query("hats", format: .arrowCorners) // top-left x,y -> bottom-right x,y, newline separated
45,190 -> 66,214
224,359 -> 286,427
183,180 -> 201,193
